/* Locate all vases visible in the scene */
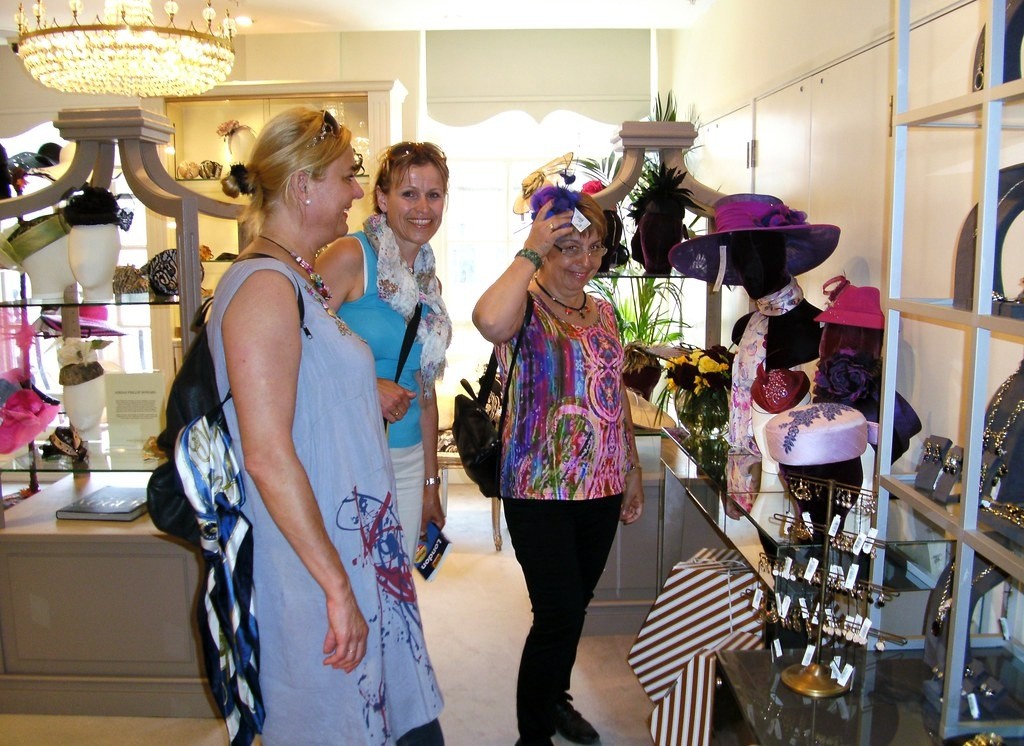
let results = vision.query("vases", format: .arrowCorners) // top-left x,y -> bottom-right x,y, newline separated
675,389 -> 732,440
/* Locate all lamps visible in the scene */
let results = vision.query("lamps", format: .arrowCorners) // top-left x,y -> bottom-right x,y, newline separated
8,0 -> 235,99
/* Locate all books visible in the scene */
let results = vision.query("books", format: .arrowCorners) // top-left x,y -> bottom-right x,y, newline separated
414,521 -> 453,583
56,487 -> 149,521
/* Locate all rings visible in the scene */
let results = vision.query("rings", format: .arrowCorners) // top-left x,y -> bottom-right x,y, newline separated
348,650 -> 356,653
550,224 -> 554,231
393,410 -> 400,416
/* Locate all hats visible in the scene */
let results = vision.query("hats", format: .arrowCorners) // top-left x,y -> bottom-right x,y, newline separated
40,305 -> 127,338
669,194 -> 841,294
0,389 -> 60,455
813,275 -> 885,329
750,360 -> 810,414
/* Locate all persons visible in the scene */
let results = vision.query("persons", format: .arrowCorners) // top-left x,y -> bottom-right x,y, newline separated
216,120 -> 257,162
597,197 -> 683,278
206,106 -> 446,746
0,186 -> 135,301
471,186 -> 645,746
731,234 -> 922,650
314,142 -> 453,574
57,340 -> 106,441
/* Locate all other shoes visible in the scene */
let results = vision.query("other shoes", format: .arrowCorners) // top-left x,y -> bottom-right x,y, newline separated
555,692 -> 600,745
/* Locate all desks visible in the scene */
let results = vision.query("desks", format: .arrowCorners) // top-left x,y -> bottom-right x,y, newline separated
718,646 -> 1024,746
656,425 -> 995,635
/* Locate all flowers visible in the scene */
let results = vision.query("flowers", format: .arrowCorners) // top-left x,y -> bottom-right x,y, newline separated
812,347 -> 883,406
216,119 -> 239,142
753,203 -> 809,228
580,180 -> 603,196
651,345 -> 733,435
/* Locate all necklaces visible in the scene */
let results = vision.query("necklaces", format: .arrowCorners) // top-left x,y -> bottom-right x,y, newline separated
403,258 -> 413,273
535,275 -> 590,320
982,372 -> 1024,454
258,235 -> 333,301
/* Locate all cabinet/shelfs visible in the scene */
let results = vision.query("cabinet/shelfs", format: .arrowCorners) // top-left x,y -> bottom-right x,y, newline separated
0,107 -> 257,721
865,0 -> 1023,739
140,78 -> 410,394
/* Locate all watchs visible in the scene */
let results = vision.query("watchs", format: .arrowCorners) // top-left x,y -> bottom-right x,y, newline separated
424,477 -> 440,486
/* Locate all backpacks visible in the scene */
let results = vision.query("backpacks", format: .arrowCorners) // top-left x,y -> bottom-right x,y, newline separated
147,253 -> 306,544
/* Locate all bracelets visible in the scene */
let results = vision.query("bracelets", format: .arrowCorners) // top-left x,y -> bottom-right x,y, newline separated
630,462 -> 642,470
514,249 -> 542,271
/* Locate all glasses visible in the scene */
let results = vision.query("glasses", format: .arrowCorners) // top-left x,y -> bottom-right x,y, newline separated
554,243 -> 608,258
305,110 -> 340,149
381,141 -> 447,182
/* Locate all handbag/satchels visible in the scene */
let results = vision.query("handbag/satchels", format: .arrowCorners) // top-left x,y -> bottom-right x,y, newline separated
452,376 -> 503,500
